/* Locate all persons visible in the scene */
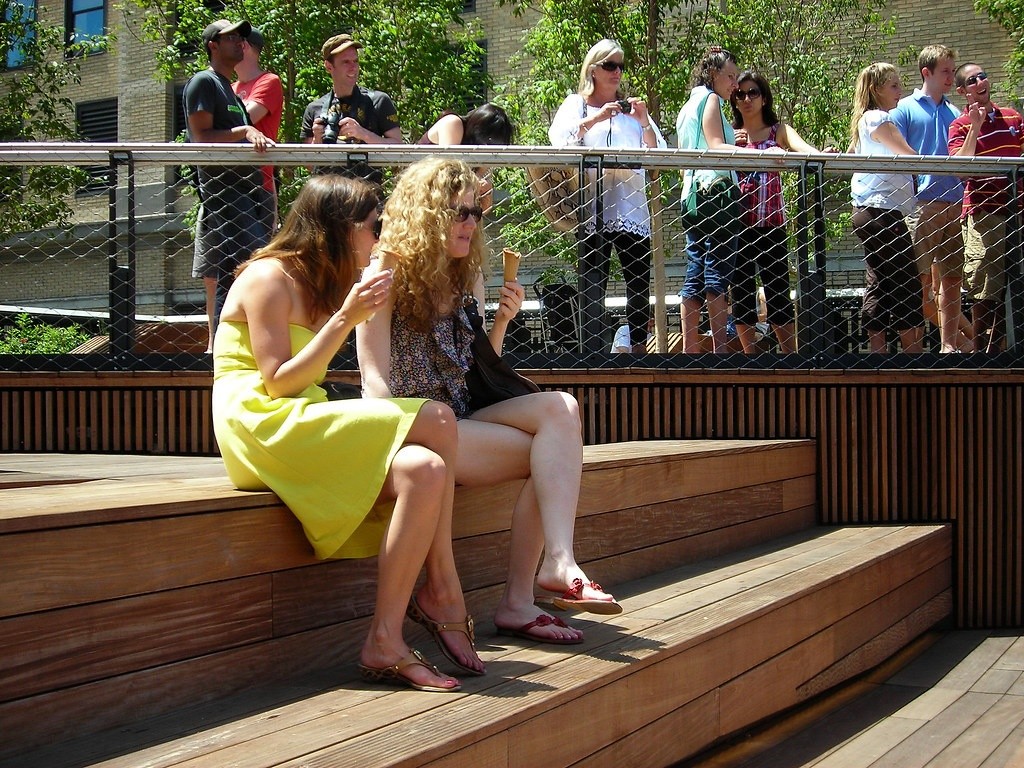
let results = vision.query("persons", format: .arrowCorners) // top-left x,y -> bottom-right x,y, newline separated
729,71 -> 840,354
676,45 -> 787,353
182,18 -> 515,353
610,310 -> 656,353
212,173 -> 487,692
548,39 -> 667,353
846,44 -> 1024,353
356,156 -> 624,644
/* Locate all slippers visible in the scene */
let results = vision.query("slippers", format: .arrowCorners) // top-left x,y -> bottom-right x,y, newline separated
533,578 -> 623,614
496,615 -> 584,644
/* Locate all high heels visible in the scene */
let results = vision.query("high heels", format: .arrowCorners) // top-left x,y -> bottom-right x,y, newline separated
406,590 -> 487,675
357,646 -> 462,692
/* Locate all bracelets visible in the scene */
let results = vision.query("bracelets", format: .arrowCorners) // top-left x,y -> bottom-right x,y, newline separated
641,123 -> 652,131
582,119 -> 590,132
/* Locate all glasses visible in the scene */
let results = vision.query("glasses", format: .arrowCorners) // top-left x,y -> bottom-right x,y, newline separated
588,61 -> 624,73
451,205 -> 483,224
363,218 -> 383,241
734,88 -> 761,102
963,72 -> 988,88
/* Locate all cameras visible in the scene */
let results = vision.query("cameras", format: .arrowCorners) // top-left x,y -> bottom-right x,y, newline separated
317,111 -> 352,145
615,101 -> 631,113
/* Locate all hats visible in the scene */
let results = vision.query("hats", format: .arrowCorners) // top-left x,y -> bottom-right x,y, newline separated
321,34 -> 363,59
247,27 -> 264,55
201,18 -> 252,48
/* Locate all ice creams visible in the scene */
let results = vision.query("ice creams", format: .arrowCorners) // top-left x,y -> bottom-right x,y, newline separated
365,249 -> 402,325
502,247 -> 521,315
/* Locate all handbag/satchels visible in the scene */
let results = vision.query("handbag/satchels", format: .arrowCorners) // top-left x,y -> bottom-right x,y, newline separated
465,298 -> 542,415
682,177 -> 743,238
526,166 -> 581,234
